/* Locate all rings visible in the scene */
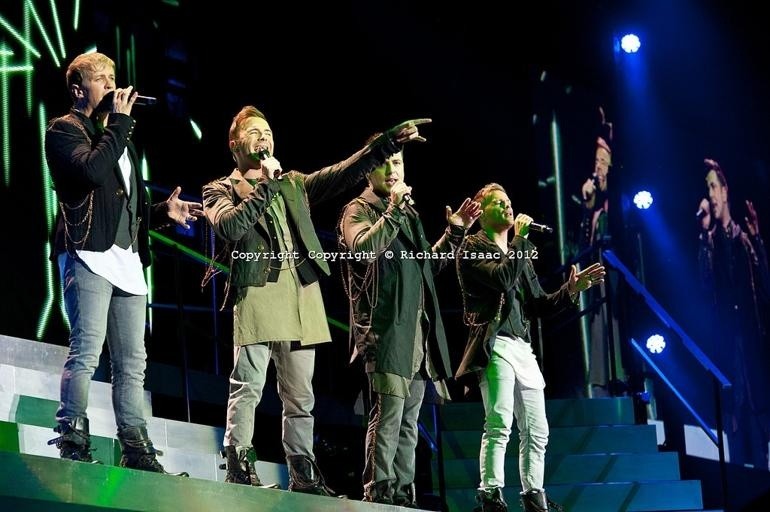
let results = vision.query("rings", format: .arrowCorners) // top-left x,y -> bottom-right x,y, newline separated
587,274 -> 591,277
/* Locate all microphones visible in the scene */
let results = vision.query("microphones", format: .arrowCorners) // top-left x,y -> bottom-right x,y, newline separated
256,145 -> 285,183
695,199 -> 714,220
105,90 -> 160,108
585,173 -> 601,203
402,194 -> 416,208
528,223 -> 554,234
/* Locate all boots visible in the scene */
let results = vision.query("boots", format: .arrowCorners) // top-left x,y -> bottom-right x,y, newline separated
393,482 -> 420,508
117,427 -> 189,477
520,493 -> 565,512
219,445 -> 281,489
48,417 -> 104,465
285,454 -> 348,498
475,488 -> 508,512
362,480 -> 395,504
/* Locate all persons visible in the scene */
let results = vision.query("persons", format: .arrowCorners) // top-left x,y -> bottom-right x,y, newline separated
694,157 -> 769,393
45,51 -> 205,478
458,182 -> 606,511
339,130 -> 483,509
202,105 -> 433,498
579,136 -> 631,399
